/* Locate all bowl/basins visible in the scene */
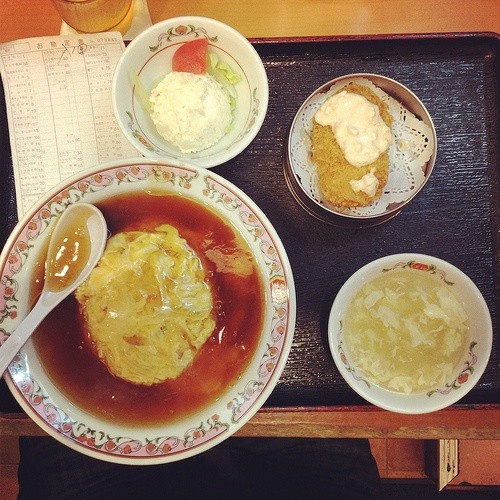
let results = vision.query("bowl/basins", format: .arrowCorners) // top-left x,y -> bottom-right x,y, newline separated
111,15 -> 270,169
328,253 -> 493,415
0,157 -> 297,466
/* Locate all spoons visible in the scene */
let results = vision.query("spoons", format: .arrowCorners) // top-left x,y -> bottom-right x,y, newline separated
0,203 -> 108,378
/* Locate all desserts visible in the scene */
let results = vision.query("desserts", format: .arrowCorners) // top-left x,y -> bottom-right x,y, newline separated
149,37 -> 242,154
